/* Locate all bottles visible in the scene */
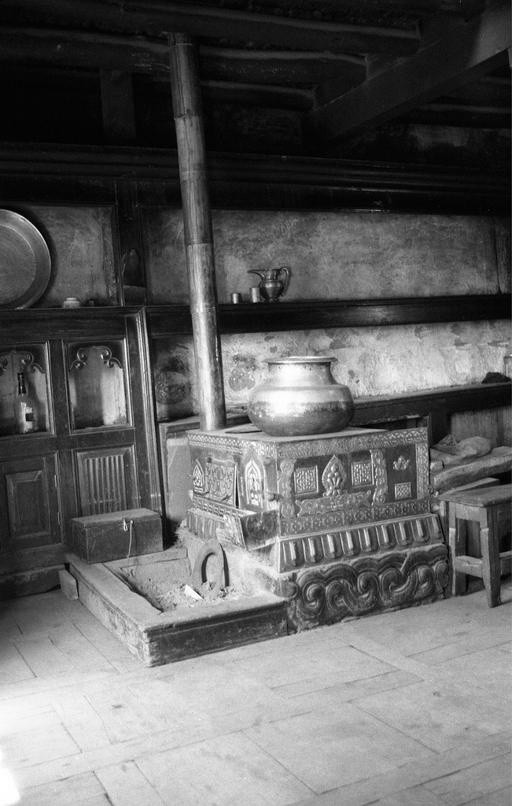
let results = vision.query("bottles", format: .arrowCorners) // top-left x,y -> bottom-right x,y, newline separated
14,371 -> 39,435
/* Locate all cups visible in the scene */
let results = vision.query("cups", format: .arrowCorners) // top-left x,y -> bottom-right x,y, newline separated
230,293 -> 242,305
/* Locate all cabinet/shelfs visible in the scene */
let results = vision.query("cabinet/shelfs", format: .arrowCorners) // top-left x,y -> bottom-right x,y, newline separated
0,307 -> 151,575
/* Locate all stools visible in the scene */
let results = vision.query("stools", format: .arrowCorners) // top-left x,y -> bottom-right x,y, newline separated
439,484 -> 512,608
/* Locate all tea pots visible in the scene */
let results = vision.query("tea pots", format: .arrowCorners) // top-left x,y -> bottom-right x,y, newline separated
248,267 -> 289,303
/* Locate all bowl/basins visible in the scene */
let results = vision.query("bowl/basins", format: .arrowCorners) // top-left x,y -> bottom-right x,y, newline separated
61,297 -> 81,309
